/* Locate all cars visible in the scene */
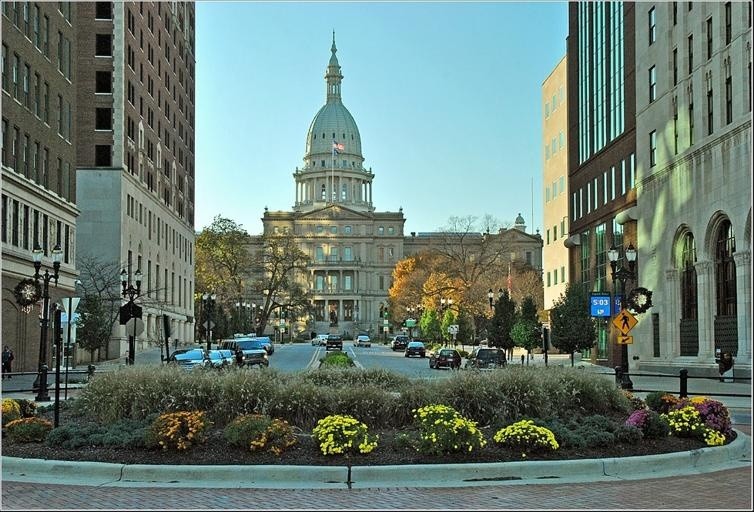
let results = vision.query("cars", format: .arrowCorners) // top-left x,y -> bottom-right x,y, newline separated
428,347 -> 461,370
203,349 -> 228,370
253,336 -> 274,355
217,350 -> 237,369
310,334 -> 328,346
164,348 -> 212,371
479,339 -> 487,345
404,341 -> 426,357
352,335 -> 371,348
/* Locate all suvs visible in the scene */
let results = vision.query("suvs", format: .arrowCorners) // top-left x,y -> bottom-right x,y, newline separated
464,344 -> 507,369
220,338 -> 269,369
390,335 -> 411,351
326,334 -> 342,351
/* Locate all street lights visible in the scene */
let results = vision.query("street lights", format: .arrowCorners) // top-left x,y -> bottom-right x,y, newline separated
406,298 -> 428,340
439,296 -> 452,346
27,241 -> 63,402
119,268 -> 144,365
234,300 -> 263,324
200,290 -> 216,350
606,242 -> 638,389
486,287 -> 503,313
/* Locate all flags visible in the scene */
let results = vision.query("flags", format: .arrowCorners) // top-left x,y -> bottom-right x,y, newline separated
332,140 -> 345,155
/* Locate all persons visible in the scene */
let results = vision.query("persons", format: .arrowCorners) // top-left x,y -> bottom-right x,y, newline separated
235,346 -> 243,367
330,309 -> 336,322
203,352 -> 228,368
2,346 -> 15,379
